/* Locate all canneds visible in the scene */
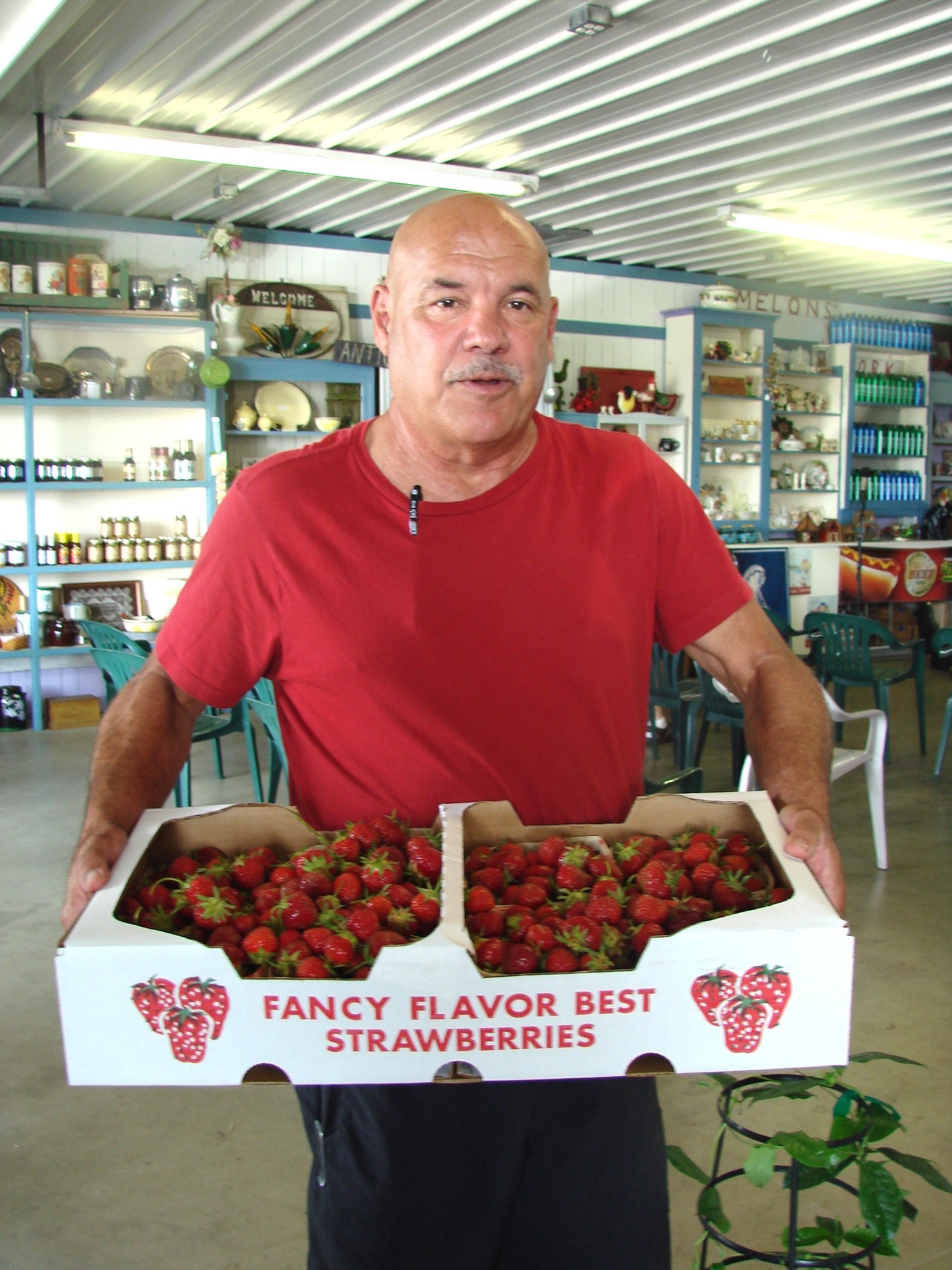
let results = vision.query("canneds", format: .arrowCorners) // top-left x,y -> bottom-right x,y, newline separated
87,514 -> 203,563
0,542 -> 26,567
0,457 -> 103,483
67,258 -> 89,296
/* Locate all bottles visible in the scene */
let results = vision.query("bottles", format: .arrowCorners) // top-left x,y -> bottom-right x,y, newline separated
0,261 -> 34,293
233,401 -> 272,431
747,421 -> 757,436
37,532 -> 83,566
173,438 -> 195,480
123,447 -> 136,482
148,447 -> 172,481
776,506 -> 790,527
781,463 -> 793,487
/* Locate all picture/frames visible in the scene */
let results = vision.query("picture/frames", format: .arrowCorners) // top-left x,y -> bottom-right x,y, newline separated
62,581 -> 142,632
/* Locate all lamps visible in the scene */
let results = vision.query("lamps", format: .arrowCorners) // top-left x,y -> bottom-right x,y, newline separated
52,117 -> 539,196
718,207 -> 952,263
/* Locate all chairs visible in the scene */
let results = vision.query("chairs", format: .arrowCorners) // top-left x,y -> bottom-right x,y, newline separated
77,618 -> 290,806
646,611 -> 951,871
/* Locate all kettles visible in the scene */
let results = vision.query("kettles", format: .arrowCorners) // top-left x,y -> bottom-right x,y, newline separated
60,603 -> 91,621
128,275 -> 155,309
164,273 -> 197,312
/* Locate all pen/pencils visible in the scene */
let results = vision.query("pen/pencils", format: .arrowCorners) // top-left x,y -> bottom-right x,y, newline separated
408,485 -> 423,537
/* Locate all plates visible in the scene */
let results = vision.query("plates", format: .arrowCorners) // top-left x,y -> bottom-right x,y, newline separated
803,460 -> 827,490
254,382 -> 311,431
799,426 -> 822,451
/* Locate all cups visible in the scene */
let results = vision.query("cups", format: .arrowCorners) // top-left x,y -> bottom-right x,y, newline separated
745,453 -> 759,463
36,262 -> 67,295
715,446 -> 726,463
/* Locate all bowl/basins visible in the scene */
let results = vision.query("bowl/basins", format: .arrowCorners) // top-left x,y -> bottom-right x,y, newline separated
199,356 -> 230,390
736,511 -> 754,520
700,281 -> 738,310
314,416 -> 342,432
122,616 -> 164,633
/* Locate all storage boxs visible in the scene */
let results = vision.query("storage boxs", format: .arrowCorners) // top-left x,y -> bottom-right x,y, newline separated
56,788 -> 855,1088
45,695 -> 100,731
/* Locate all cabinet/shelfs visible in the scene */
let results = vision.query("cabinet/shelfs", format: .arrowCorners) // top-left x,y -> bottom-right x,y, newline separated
0,318 -> 375,733
378,307 -> 951,540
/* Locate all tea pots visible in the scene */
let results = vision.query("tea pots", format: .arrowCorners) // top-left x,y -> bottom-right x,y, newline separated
211,294 -> 246,356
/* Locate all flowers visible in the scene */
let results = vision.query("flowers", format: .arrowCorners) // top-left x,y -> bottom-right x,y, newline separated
202,223 -> 241,259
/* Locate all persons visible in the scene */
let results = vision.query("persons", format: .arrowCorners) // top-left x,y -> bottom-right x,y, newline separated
63,193 -> 845,1270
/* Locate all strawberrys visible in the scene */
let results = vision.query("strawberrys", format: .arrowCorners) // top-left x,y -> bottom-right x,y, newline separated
464,823 -> 792,976
115,809 -> 443,981
